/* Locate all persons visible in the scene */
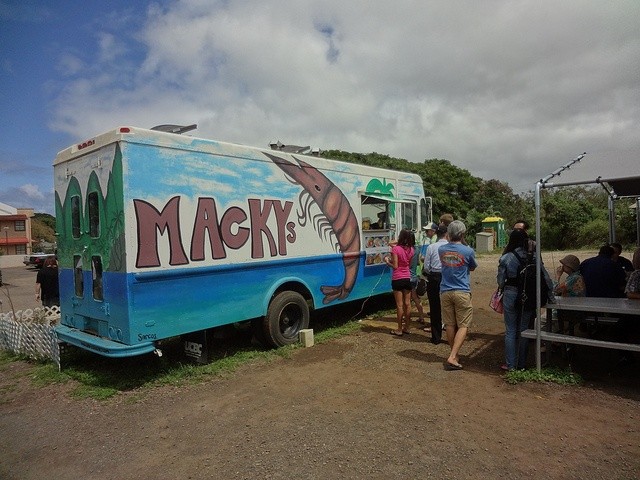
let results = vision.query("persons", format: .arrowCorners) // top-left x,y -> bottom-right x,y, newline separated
420,223 -> 438,317
609,243 -> 634,281
551,255 -> 591,337
424,213 -> 455,332
502,222 -> 546,340
581,246 -> 628,299
406,234 -> 425,322
437,221 -> 478,368
496,229 -> 535,371
76,256 -> 97,296
36,257 -> 59,324
383,228 -> 416,335
425,224 -> 459,344
624,248 -> 640,299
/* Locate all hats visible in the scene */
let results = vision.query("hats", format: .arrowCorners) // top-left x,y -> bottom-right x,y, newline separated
559,255 -> 580,272
422,222 -> 438,231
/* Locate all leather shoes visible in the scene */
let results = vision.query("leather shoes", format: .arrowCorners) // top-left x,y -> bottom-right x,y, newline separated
423,326 -> 432,332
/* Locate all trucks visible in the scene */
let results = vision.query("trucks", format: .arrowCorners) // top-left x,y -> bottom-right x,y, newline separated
54,123 -> 437,379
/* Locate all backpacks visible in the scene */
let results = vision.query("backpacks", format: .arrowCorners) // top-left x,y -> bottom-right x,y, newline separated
511,249 -> 552,311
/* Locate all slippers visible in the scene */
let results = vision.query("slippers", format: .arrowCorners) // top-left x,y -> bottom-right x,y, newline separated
447,362 -> 464,370
402,329 -> 411,334
390,329 -> 403,337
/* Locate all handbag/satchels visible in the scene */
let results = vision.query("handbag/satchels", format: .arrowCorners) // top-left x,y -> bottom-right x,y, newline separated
416,277 -> 427,296
489,287 -> 504,314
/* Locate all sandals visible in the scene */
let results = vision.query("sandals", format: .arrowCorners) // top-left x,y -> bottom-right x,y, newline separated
402,316 -> 412,322
416,319 -> 424,324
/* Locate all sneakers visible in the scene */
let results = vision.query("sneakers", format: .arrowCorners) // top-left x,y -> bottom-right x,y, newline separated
499,362 -> 516,372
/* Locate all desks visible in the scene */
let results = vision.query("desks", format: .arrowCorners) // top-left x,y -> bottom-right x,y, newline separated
545,294 -> 640,337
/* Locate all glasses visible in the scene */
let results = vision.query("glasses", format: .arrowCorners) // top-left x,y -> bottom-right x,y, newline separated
425,230 -> 431,232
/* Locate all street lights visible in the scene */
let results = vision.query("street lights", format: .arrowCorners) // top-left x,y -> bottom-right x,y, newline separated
3,227 -> 10,255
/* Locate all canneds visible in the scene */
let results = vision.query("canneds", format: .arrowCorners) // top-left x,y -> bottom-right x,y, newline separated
383,222 -> 386,229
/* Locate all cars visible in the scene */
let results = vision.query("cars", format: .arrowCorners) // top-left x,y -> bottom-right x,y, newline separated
22,248 -> 57,271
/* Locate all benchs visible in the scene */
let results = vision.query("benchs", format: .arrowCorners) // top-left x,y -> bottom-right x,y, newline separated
521,329 -> 640,375
